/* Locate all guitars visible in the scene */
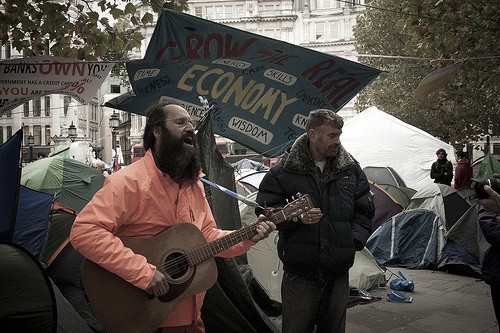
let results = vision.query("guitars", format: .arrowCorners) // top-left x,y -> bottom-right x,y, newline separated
78,192 -> 314,333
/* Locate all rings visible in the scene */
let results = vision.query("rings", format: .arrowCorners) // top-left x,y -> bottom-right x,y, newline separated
308,214 -> 310,217
309,222 -> 311,224
309,219 -> 312,221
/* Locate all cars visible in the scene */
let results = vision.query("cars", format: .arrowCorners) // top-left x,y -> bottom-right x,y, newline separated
234,170 -> 270,218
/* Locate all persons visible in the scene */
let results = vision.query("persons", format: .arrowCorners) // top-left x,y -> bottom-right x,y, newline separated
454,151 -> 472,189
68,102 -> 277,333
476,185 -> 500,214
430,149 -> 453,186
256,109 -> 375,333
478,173 -> 500,333
38,152 -> 46,159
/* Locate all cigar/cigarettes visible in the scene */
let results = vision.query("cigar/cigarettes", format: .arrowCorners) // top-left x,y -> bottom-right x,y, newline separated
319,213 -> 322,215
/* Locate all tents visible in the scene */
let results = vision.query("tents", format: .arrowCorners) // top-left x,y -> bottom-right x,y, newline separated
340,107 -> 500,275
0,129 -> 106,333
238,191 -> 403,306
235,170 -> 267,196
231,157 -> 270,171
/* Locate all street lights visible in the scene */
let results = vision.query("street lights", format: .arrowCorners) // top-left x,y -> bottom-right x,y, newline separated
27,133 -> 35,163
108,109 -> 122,172
68,121 -> 77,144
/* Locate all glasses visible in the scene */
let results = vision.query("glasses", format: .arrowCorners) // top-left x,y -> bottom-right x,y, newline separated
162,115 -> 196,128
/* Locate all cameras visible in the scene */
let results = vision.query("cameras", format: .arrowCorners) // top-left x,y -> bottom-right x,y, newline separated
469,177 -> 500,200
